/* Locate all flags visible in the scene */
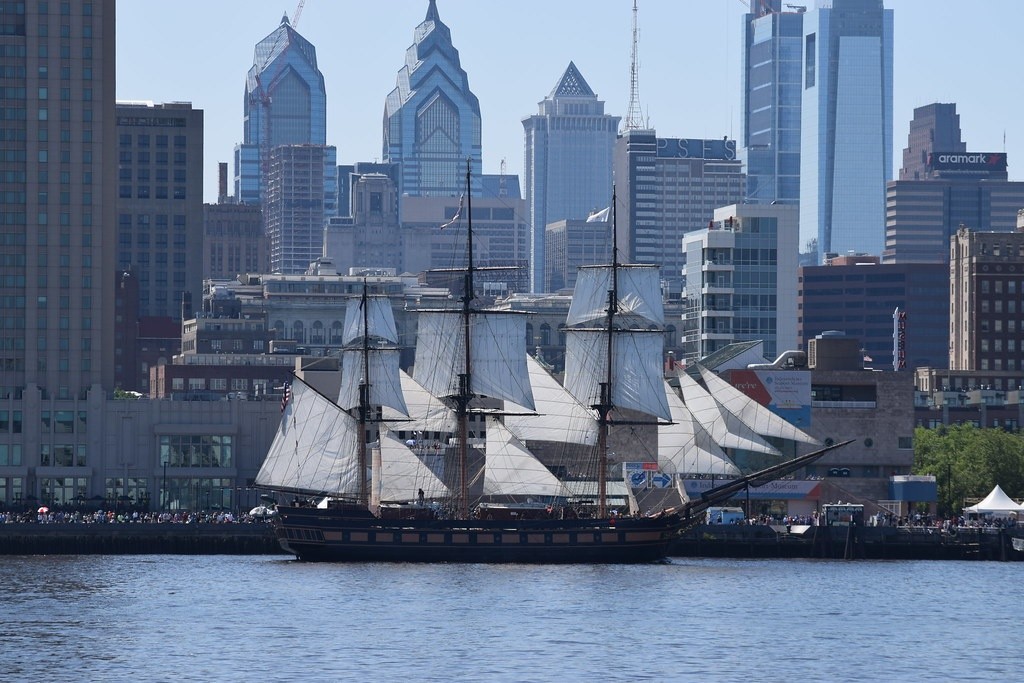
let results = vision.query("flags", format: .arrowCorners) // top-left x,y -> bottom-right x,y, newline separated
280,380 -> 291,414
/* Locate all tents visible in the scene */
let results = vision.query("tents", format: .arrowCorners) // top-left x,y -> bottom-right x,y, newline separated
962,484 -> 1024,522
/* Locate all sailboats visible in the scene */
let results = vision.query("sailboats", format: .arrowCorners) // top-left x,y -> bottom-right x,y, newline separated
244,156 -> 860,565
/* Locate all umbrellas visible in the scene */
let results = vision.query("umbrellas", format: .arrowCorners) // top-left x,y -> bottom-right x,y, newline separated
37,506 -> 49,514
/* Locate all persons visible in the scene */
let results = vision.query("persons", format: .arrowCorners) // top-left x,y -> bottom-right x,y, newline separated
418,488 -> 424,504
0,510 -> 253,523
707,512 -> 1016,527
605,508 -> 653,518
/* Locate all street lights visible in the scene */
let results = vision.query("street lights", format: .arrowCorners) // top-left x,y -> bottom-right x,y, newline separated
229,487 -> 233,512
237,486 -> 242,515
254,486 -> 258,507
220,487 -> 223,511
245,486 -> 251,514
162,461 -> 170,514
206,490 -> 210,515
